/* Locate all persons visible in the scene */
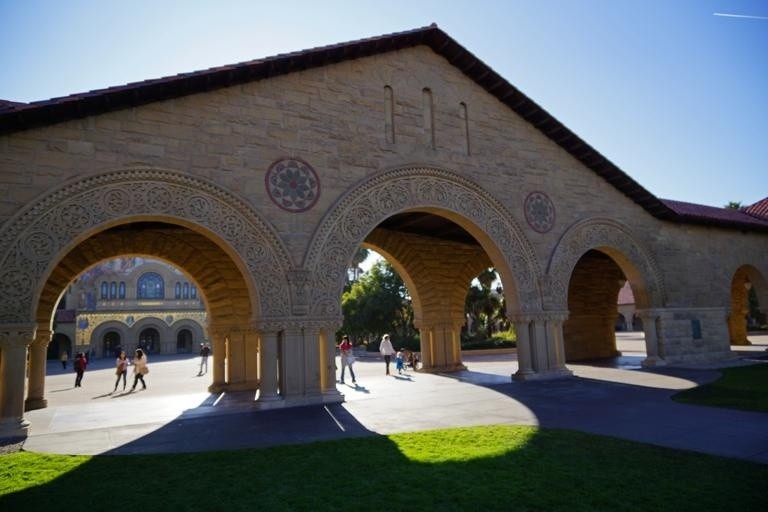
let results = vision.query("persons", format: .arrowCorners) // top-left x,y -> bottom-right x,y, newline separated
395,349 -> 408,375
403,354 -> 419,371
379,334 -> 397,375
112,351 -> 131,393
339,336 -> 356,383
130,349 -> 149,392
197,343 -> 210,375
61,350 -> 68,370
72,347 -> 96,387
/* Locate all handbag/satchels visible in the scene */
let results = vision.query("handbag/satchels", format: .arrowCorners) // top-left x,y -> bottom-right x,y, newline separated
346,356 -> 354,365
140,367 -> 148,374
